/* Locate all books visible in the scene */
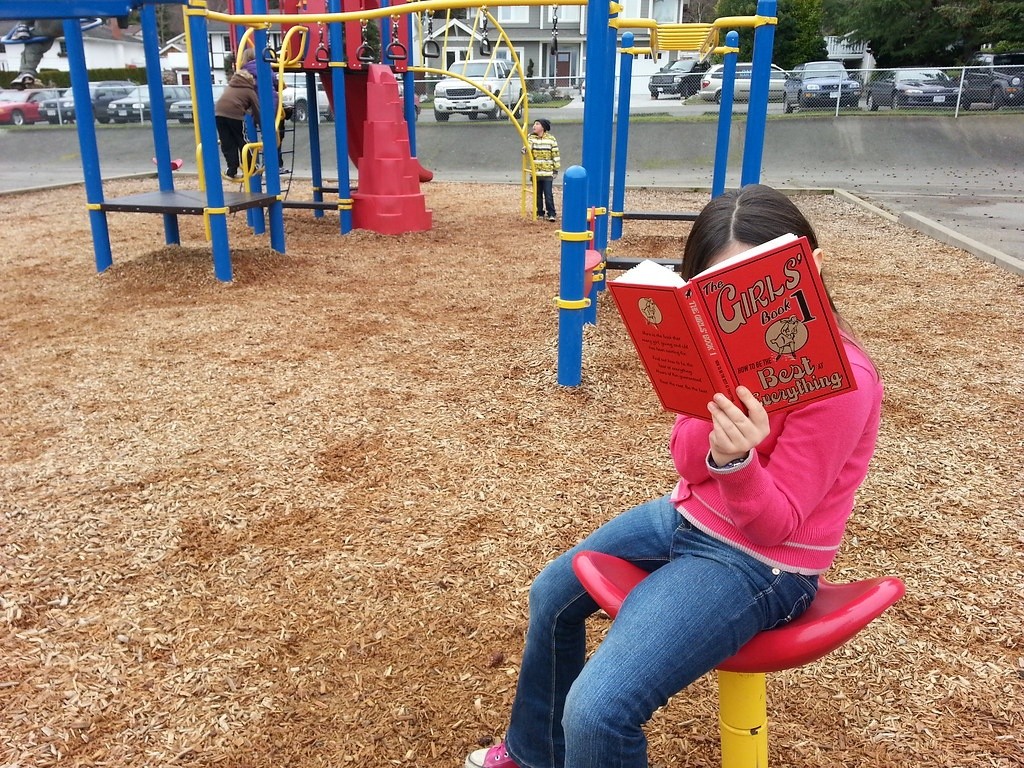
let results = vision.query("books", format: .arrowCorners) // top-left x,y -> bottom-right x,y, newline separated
603,234 -> 859,425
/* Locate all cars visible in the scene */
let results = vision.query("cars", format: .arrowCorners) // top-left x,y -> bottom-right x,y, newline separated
171,85 -> 232,122
0,88 -> 59,125
865,68 -> 967,111
277,71 -> 336,124
648,57 -> 711,100
107,83 -> 192,123
398,80 -> 422,122
779,61 -> 862,113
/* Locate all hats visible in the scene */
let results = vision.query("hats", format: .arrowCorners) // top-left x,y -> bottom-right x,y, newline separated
534,119 -> 550,131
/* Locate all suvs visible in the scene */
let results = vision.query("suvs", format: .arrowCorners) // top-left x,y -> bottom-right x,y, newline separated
40,79 -> 140,123
432,59 -> 523,122
961,50 -> 1024,109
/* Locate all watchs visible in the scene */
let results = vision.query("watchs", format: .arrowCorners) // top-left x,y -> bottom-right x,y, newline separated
709,456 -> 747,471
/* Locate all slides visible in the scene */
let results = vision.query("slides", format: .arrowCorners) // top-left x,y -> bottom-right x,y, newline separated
321,66 -> 434,183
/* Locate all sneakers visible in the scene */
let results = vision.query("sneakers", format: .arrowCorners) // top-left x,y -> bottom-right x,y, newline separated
465,742 -> 521,768
279,167 -> 292,174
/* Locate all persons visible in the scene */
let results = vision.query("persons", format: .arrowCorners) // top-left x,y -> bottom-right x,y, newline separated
240,49 -> 291,176
1,18 -> 63,90
215,67 -> 264,180
464,183 -> 882,768
521,118 -> 560,221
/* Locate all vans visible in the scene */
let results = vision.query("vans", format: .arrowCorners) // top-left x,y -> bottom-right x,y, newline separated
702,62 -> 795,106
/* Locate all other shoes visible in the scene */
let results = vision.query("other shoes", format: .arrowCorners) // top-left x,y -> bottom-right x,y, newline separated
549,215 -> 555,221
537,215 -> 543,220
